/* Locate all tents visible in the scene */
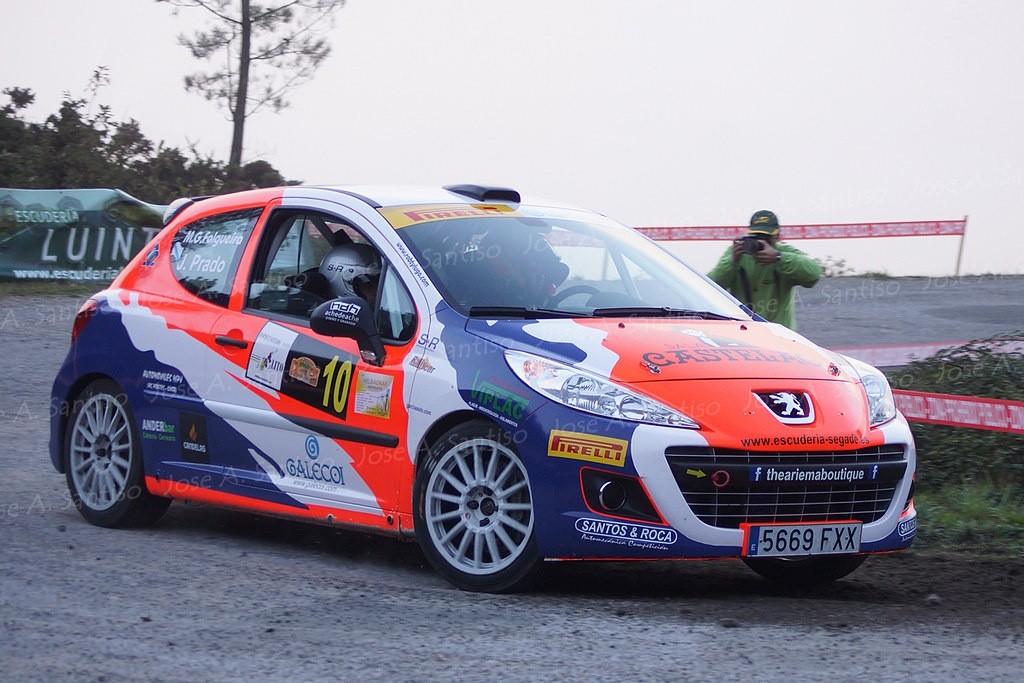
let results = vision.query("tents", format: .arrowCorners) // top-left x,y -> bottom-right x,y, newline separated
0,187 -> 171,284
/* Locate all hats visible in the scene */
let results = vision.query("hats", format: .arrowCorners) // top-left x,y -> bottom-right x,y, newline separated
746,210 -> 780,235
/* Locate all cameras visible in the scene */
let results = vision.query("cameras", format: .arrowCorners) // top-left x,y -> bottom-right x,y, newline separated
743,236 -> 761,253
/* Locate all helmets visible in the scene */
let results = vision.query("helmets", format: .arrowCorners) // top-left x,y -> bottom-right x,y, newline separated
320,242 -> 381,299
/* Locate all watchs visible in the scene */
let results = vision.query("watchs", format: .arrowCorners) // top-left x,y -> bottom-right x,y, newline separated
774,251 -> 781,263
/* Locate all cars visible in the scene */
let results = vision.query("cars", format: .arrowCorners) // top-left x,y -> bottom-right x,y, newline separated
47,180 -> 918,597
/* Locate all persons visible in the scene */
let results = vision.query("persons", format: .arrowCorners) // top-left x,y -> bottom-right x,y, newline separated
706,211 -> 825,333
471,230 -> 566,309
305,244 -> 415,342
